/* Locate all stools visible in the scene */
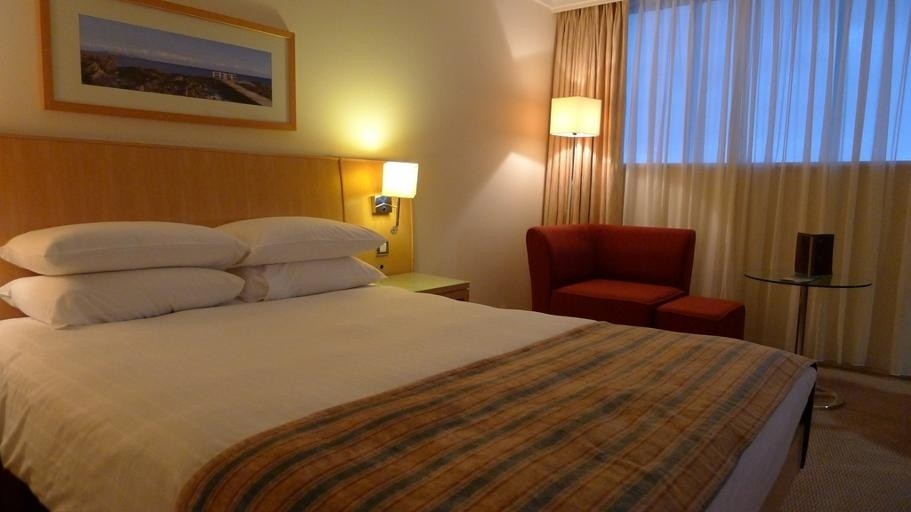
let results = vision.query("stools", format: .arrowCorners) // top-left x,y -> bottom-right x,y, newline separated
658,289 -> 746,336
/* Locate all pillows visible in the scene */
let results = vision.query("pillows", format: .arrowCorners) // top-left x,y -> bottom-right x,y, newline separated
0,268 -> 247,327
239,257 -> 396,304
1,221 -> 246,276
218,221 -> 388,258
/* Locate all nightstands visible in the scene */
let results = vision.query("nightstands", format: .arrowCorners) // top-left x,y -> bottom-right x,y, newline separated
375,273 -> 474,303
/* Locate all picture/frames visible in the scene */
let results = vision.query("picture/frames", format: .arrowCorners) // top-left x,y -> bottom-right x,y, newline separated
39,0 -> 297,132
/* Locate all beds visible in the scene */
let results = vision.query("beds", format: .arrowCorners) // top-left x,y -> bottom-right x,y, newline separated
1,132 -> 819,511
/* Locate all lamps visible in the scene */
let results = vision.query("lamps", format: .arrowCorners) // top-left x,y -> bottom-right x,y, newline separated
371,161 -> 418,233
550,92 -> 602,223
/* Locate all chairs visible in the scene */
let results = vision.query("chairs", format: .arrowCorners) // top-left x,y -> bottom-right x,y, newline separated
525,224 -> 697,318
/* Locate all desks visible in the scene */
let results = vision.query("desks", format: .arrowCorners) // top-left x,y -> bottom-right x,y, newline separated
746,264 -> 871,413
